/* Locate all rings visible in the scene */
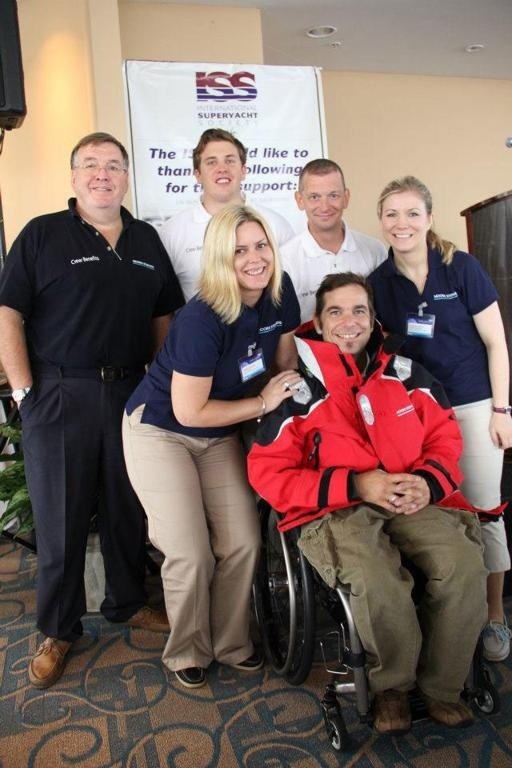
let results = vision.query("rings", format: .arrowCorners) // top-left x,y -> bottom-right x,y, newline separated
388,492 -> 402,507
282,378 -> 291,392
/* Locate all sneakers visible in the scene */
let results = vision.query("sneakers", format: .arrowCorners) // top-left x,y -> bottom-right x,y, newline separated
210,627 -> 265,672
28,621 -> 76,690
480,604 -> 512,662
415,674 -> 475,731
169,636 -> 207,689
104,595 -> 175,634
369,667 -> 414,738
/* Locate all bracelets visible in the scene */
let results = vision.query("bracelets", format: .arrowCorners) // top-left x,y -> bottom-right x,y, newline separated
252,392 -> 267,425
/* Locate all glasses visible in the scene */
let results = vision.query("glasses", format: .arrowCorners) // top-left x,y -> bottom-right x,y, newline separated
71,163 -> 129,176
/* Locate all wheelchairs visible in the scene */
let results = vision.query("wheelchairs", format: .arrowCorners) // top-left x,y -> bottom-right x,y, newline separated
233,356 -> 501,750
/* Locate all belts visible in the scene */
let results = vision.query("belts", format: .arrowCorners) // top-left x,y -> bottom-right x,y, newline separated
33,363 -> 151,383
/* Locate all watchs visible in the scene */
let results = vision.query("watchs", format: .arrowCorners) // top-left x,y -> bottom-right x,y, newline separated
9,387 -> 34,402
490,404 -> 512,414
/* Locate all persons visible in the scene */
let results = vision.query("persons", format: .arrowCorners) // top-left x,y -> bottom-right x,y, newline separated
365,173 -> 512,661
155,127 -> 295,307
247,271 -> 492,736
0,131 -> 190,691
276,158 -> 389,324
119,201 -> 301,688
